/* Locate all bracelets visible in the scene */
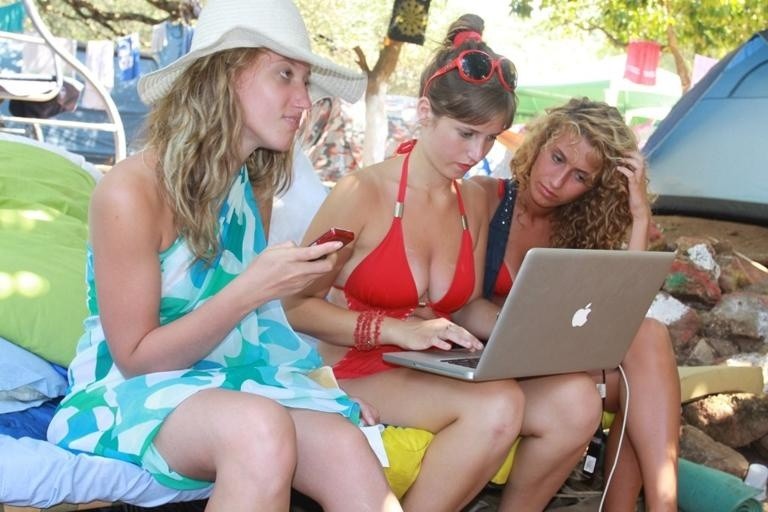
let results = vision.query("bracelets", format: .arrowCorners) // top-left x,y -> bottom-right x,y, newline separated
354,309 -> 388,351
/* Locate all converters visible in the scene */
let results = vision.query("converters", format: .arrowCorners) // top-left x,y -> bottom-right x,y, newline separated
582,430 -> 602,477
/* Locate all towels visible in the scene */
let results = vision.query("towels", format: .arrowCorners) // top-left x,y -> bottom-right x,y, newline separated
117,30 -> 139,87
81,39 -> 114,109
622,39 -> 657,90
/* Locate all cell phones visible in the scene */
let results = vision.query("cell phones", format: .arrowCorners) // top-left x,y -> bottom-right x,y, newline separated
316,228 -> 354,250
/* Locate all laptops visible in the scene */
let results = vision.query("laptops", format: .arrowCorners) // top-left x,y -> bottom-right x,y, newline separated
382,248 -> 677,383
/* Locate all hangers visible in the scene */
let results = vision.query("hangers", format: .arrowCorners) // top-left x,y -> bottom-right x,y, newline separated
161,7 -> 182,32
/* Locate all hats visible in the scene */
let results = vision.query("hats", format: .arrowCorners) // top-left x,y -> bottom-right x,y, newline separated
138,0 -> 368,112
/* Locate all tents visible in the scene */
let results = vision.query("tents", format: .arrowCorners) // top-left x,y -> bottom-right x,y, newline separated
639,27 -> 767,230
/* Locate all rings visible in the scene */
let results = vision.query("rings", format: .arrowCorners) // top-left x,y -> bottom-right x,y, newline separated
447,323 -> 452,329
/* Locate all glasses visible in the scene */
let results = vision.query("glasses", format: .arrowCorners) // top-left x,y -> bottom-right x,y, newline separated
421,49 -> 519,97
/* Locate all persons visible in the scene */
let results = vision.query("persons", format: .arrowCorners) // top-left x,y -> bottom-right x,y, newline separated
467,93 -> 684,512
42,0 -> 406,512
281,13 -> 602,512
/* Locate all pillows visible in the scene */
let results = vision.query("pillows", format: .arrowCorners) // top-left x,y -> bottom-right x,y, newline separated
0,338 -> 73,421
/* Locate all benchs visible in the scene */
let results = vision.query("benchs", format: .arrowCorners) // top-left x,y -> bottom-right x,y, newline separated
0,1 -> 762,512
